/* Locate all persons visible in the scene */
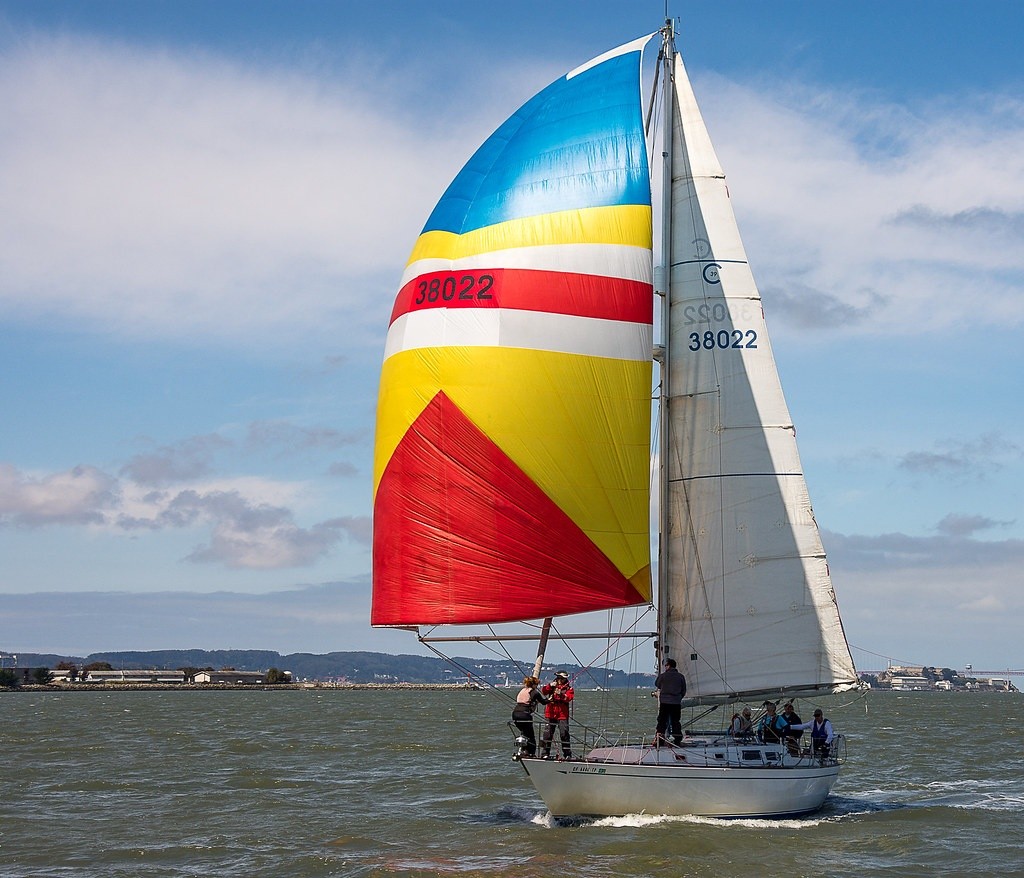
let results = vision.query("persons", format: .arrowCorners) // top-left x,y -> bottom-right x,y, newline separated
734,706 -> 754,737
511,676 -> 559,759
540,670 -> 575,760
757,702 -> 789,745
650,659 -> 687,747
789,709 -> 834,760
728,713 -> 740,735
780,703 -> 804,745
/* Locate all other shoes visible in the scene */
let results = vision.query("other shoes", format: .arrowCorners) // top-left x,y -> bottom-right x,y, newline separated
542,756 -> 548,759
566,756 -> 570,759
653,741 -> 665,747
669,742 -> 680,747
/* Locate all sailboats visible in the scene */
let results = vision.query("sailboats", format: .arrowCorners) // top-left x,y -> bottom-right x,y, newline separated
371,0 -> 870,823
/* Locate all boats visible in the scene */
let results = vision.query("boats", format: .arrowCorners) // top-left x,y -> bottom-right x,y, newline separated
892,676 -> 929,690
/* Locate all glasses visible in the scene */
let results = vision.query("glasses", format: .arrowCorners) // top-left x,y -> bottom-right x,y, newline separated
813,715 -> 820,717
745,714 -> 751,717
665,664 -> 668,667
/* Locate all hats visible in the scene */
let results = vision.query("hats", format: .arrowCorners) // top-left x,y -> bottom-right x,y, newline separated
814,709 -> 823,714
554,670 -> 570,679
743,706 -> 752,715
